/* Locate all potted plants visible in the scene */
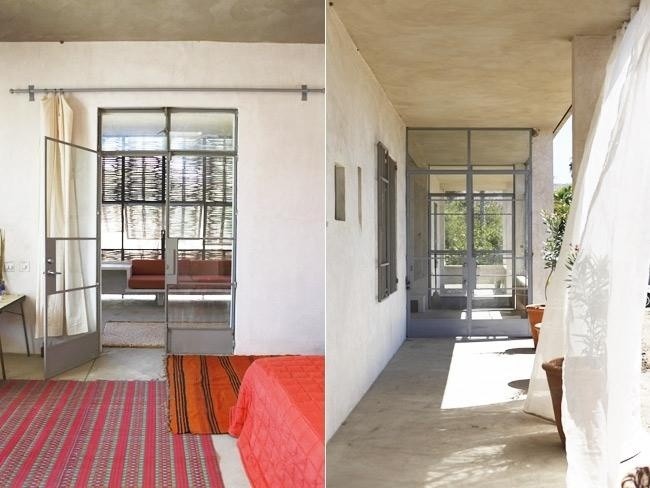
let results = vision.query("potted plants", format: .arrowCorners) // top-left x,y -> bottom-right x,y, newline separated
526,184 -> 609,452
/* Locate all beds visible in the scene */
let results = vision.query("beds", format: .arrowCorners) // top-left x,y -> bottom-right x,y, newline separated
236,354 -> 324,488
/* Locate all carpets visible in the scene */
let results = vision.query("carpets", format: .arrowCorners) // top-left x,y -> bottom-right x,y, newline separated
102,320 -> 229,350
0,353 -> 302,488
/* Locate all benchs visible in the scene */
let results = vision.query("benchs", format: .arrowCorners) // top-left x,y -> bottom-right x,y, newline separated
126,259 -> 231,306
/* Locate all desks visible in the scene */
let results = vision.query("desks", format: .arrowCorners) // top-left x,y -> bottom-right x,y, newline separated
0,293 -> 31,381
